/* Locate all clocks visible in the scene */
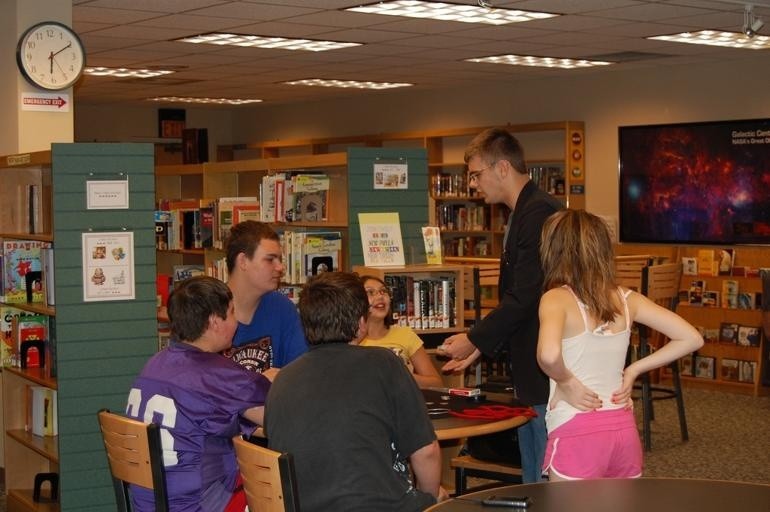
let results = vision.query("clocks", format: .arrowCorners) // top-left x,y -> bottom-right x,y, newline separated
16,20 -> 87,92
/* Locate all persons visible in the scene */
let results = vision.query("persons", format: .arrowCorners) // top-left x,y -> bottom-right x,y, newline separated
125,274 -> 275,511
438,127 -> 568,483
356,273 -> 444,388
265,272 -> 448,511
216,220 -> 310,385
536,209 -> 705,482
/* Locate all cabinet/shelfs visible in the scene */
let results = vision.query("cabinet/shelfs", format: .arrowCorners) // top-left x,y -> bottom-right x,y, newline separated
659,246 -> 770,396
259,131 -> 425,159
203,149 -> 429,309
0,143 -> 157,511
425,121 -> 586,263
354,266 -> 480,389
156,165 -> 203,351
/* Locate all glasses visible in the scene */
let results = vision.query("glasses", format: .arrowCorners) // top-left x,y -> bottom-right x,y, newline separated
470,164 -> 494,181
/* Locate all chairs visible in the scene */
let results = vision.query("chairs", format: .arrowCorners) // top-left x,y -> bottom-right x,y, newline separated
232,433 -> 300,512
97,408 -> 169,512
449,457 -> 522,494
630,262 -> 689,450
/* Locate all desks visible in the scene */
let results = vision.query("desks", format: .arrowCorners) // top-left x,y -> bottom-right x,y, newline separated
419,388 -> 534,439
421,477 -> 769,512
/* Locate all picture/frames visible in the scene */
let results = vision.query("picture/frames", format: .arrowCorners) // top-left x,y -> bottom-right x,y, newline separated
81,232 -> 137,304
85,180 -> 129,210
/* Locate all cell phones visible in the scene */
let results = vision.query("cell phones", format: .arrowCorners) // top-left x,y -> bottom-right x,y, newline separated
482,495 -> 531,507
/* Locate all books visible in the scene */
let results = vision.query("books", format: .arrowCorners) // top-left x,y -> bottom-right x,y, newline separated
432,165 -> 564,257
630,249 -> 770,384
382,276 -> 456,329
1,239 -> 57,439
157,169 -> 343,332
